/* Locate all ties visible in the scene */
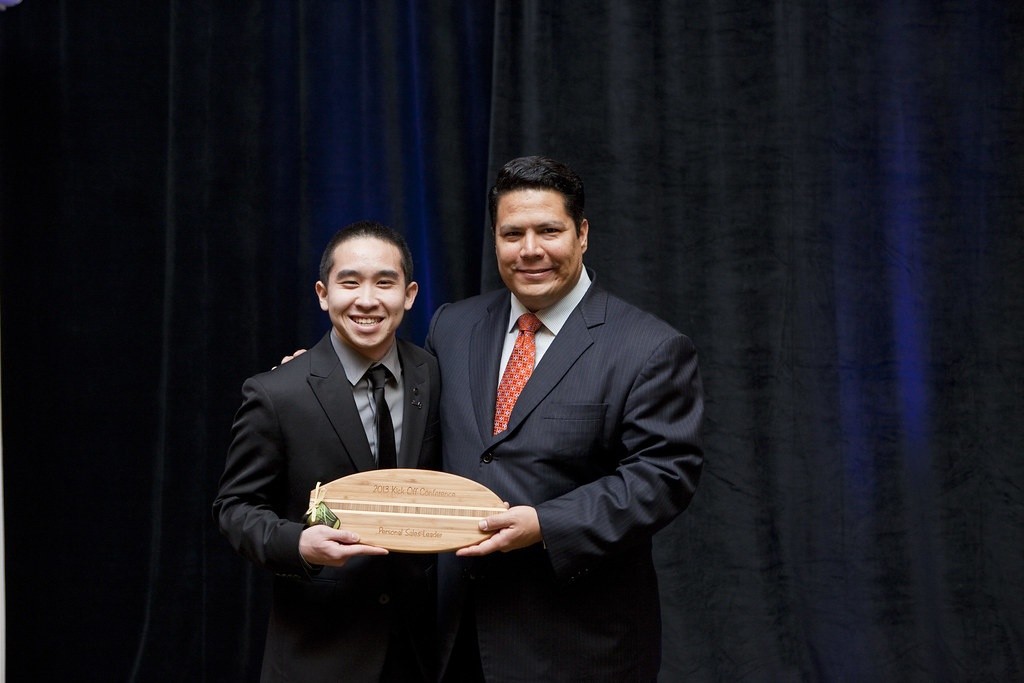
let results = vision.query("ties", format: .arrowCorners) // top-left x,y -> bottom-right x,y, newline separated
493,313 -> 544,436
367,364 -> 397,468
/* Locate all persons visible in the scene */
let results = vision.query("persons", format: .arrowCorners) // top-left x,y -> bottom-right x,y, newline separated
212,220 -> 440,683
270,154 -> 706,683
